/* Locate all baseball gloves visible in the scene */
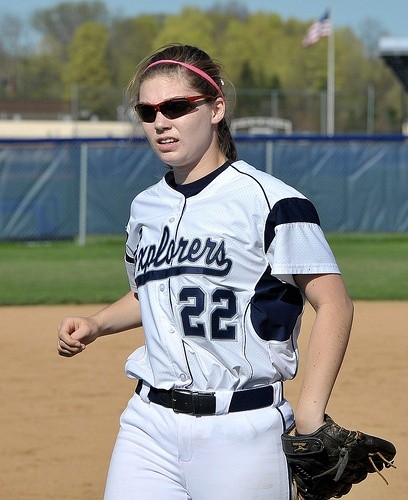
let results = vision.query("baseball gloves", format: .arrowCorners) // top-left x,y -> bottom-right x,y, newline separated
278,413 -> 396,500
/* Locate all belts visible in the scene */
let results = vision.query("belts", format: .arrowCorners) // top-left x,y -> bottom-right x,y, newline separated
135,378 -> 283,416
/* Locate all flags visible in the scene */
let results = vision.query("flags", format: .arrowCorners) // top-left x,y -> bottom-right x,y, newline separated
300,7 -> 332,49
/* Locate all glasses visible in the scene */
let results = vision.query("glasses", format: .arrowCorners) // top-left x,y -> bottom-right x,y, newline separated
134,95 -> 215,123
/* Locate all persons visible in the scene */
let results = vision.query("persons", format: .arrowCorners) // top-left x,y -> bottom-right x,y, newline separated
57,45 -> 355,500
